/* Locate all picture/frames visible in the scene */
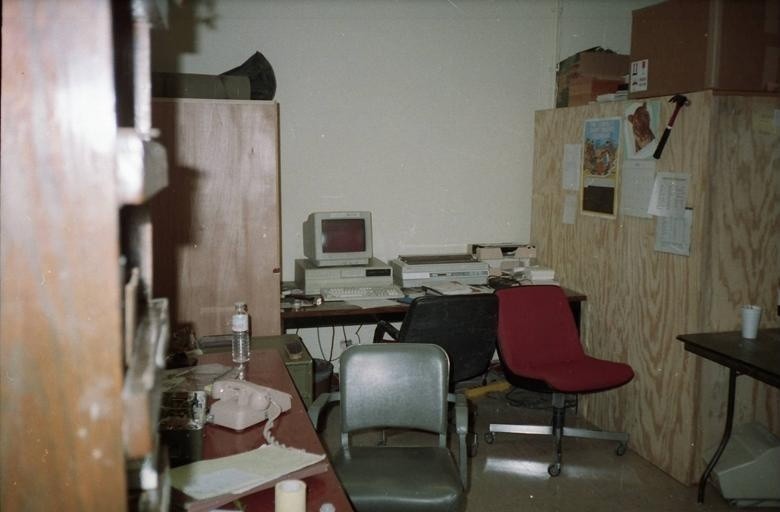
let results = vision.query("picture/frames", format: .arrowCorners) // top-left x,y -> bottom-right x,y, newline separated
580,116 -> 623,221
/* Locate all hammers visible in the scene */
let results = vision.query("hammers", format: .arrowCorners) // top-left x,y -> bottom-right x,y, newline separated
653,93 -> 690,159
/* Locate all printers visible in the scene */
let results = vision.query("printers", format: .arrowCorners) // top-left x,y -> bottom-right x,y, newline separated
388,255 -> 490,288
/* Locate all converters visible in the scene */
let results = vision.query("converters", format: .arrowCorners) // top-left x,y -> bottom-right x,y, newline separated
492,277 -> 513,288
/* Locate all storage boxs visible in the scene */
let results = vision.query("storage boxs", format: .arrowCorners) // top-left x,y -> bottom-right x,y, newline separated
554,0 -> 780,110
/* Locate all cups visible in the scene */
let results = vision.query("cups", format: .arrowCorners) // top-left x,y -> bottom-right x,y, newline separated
740,304 -> 763,341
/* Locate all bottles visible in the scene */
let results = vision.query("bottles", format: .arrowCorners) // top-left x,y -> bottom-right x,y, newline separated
232,302 -> 251,364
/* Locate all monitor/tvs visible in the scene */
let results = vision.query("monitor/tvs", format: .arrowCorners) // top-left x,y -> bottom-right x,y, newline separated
303,211 -> 373,267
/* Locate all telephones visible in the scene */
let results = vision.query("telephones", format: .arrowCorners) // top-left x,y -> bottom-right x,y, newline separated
206,379 -> 292,430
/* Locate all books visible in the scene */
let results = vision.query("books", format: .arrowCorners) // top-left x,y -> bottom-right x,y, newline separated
421,280 -> 496,296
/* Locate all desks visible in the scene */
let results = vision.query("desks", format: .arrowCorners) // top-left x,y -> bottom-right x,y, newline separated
280,280 -> 588,344
675,328 -> 780,505
159,347 -> 355,511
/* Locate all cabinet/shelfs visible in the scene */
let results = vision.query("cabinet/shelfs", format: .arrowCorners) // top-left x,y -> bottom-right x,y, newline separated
148,95 -> 282,340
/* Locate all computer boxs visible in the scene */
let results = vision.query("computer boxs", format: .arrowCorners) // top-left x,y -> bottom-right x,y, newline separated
294,257 -> 393,295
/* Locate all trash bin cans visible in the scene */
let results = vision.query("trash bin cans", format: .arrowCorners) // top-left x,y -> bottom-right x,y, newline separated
312,359 -> 334,434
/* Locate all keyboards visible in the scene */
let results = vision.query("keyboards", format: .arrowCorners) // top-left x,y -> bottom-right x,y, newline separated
320,286 -> 405,301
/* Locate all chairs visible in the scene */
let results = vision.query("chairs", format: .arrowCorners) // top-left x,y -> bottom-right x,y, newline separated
315,344 -> 474,512
491,284 -> 636,478
370,290 -> 501,461
